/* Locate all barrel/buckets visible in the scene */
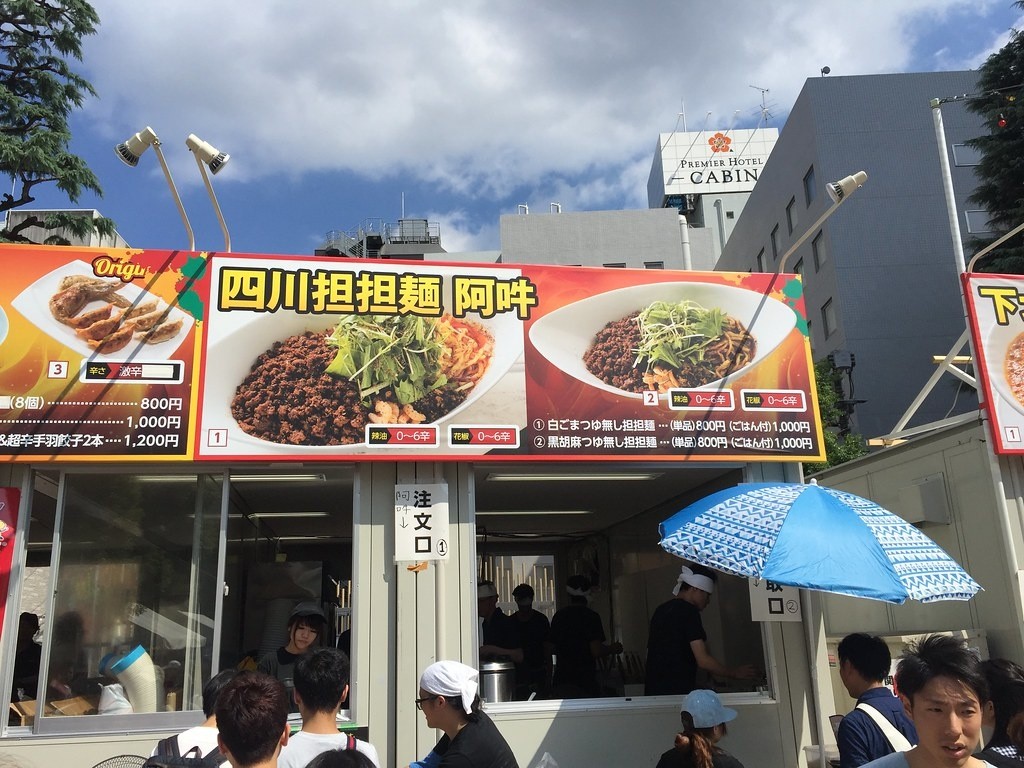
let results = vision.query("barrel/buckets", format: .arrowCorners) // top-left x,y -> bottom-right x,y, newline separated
480,654 -> 517,702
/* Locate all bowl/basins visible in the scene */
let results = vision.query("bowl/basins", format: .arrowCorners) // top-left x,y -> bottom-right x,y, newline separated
529,281 -> 798,400
202,275 -> 525,450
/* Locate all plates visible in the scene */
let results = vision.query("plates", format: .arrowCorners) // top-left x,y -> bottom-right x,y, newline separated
10,259 -> 196,361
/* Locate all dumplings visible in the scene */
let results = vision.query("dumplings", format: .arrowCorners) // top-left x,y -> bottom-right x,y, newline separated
62,297 -> 185,352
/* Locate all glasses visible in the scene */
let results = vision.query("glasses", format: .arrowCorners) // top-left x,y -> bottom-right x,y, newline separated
415,697 -> 430,710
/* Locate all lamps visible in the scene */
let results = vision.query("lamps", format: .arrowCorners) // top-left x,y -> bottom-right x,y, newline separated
186,134 -> 231,253
778,171 -> 867,274
673,109 -> 769,132
113,126 -> 195,254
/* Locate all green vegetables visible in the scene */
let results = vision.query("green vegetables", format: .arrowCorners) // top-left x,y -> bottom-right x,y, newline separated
323,309 -> 449,404
628,299 -> 729,373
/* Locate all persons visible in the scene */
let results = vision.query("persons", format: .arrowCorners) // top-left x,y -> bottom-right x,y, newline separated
216,669 -> 290,768
149,668 -> 240,768
277,645 -> 380,768
477,580 -> 528,701
644,564 -> 756,696
10,612 -> 42,703
305,748 -> 377,768
656,689 -> 744,768
970,657 -> 1024,768
550,575 -> 618,699
256,601 -> 327,713
837,633 -> 919,768
857,633 -> 998,768
402,660 -> 519,768
510,583 -> 550,700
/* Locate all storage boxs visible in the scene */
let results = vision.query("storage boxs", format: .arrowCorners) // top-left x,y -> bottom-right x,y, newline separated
10,694 -> 101,726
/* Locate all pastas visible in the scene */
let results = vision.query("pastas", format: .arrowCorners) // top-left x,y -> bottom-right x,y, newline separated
440,319 -> 491,384
702,316 -> 754,380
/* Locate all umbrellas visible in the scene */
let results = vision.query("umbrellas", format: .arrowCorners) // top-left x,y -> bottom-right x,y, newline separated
657,477 -> 986,768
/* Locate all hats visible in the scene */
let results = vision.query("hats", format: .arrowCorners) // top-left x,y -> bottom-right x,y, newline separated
512,584 -> 534,597
680,689 -> 737,728
288,600 -> 328,624
